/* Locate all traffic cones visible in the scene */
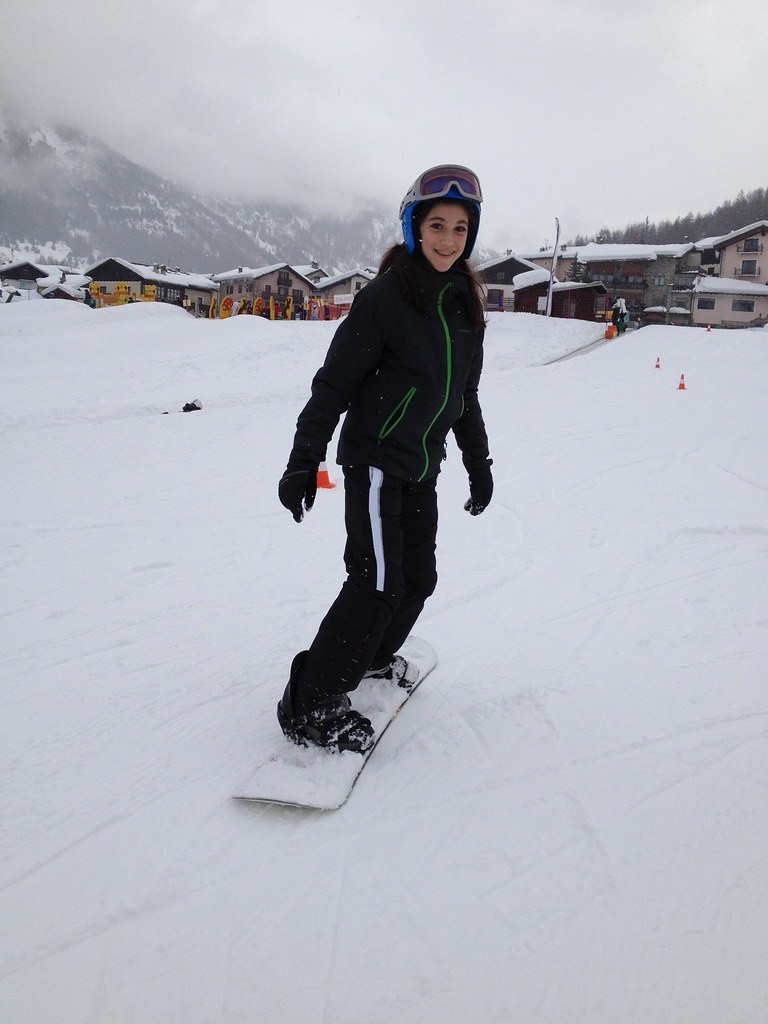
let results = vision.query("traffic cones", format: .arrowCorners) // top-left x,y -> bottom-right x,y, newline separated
706,324 -> 712,331
315,454 -> 338,488
678,374 -> 686,389
654,358 -> 660,367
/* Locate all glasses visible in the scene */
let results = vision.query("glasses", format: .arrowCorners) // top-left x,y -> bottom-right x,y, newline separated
415,162 -> 481,201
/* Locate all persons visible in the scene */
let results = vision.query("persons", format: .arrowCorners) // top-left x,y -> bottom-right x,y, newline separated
278,164 -> 494,756
323,305 -> 330,321
128,297 -> 134,303
611,295 -> 627,329
84,289 -> 96,309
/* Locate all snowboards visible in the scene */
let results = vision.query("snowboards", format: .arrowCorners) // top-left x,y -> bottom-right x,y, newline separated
227,636 -> 438,811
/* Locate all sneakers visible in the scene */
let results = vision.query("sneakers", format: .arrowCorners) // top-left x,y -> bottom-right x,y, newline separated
277,652 -> 376,753
354,650 -> 419,689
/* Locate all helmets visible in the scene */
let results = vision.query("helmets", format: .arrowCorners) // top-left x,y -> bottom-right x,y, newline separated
400,166 -> 482,259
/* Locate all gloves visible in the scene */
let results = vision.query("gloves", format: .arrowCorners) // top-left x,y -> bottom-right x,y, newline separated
278,467 -> 317,521
464,464 -> 494,516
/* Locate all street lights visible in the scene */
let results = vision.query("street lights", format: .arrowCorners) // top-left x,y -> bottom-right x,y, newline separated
544,217 -> 560,316
